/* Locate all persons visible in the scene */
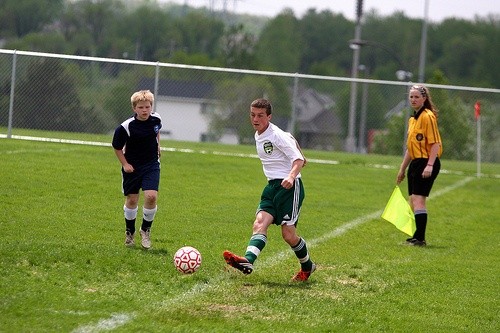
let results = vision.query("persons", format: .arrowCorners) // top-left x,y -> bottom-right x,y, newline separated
112,89 -> 162,249
223,99 -> 316,282
397,85 -> 442,246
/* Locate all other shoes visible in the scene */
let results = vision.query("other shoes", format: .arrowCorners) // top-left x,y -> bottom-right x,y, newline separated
406,238 -> 427,247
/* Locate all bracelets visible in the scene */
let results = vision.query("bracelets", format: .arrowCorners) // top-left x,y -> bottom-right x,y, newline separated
427,163 -> 433,166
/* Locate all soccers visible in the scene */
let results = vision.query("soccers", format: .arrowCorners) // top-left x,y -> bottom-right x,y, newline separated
174,246 -> 202,275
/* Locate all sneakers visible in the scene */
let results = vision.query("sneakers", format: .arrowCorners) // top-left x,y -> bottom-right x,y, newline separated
125,231 -> 135,248
291,262 -> 316,283
139,226 -> 151,249
223,250 -> 253,275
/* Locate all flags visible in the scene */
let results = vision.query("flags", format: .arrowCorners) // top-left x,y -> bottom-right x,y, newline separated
381,185 -> 416,237
